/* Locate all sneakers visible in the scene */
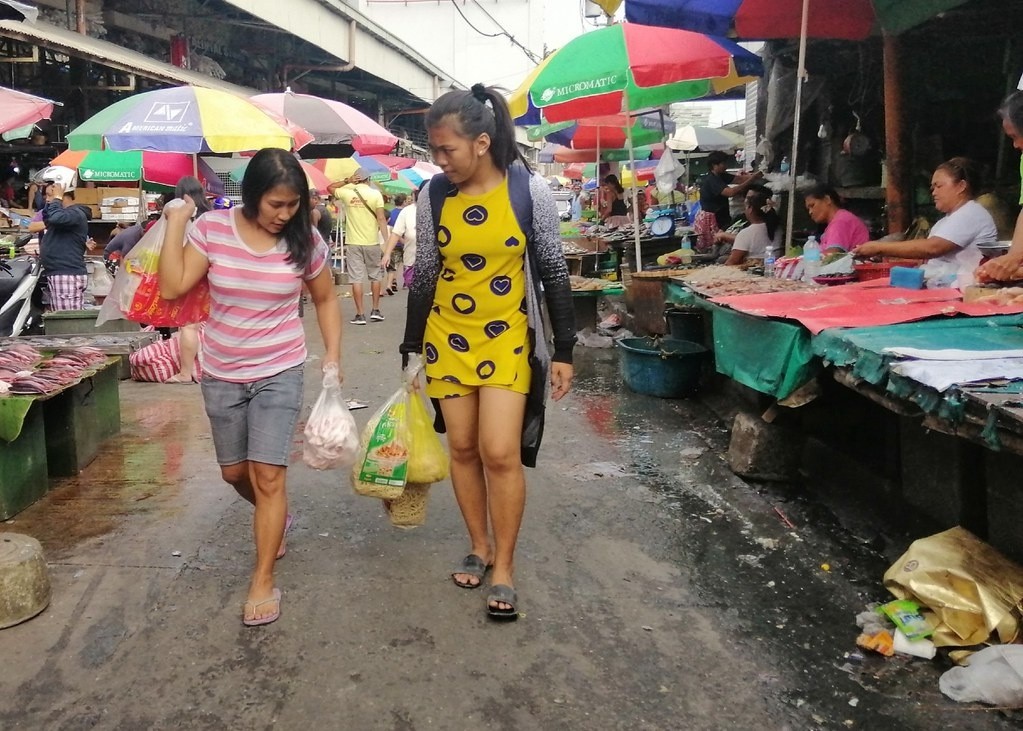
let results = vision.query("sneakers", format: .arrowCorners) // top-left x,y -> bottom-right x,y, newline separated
350,314 -> 367,325
369,309 -> 385,321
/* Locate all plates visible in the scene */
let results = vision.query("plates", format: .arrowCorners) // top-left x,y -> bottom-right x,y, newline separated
812,276 -> 855,285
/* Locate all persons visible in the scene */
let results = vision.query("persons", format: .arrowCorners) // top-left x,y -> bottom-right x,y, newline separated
160,147 -> 342,624
26,166 -> 96,315
105,173 -> 232,384
569,182 -> 588,221
628,189 -> 650,217
602,174 -> 627,219
699,150 -> 763,227
327,167 -> 389,325
306,178 -> 432,297
851,157 -> 998,289
399,82 -> 577,620
714,186 -> 783,265
804,183 -> 869,254
977,90 -> 1023,281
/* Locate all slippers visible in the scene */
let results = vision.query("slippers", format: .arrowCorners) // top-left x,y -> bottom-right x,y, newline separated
274,513 -> 293,559
244,587 -> 281,625
485,585 -> 519,620
452,553 -> 493,589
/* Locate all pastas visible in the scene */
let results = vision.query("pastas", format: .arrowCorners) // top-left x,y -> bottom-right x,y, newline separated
352,419 -> 430,527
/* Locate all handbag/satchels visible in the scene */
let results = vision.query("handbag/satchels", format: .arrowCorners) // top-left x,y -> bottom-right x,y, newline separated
384,484 -> 430,530
351,353 -> 427,501
407,388 -> 448,484
303,361 -> 358,471
93,198 -> 212,326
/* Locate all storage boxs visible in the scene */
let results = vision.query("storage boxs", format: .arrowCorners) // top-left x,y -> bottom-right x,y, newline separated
98,187 -> 140,220
73,188 -> 102,204
83,204 -> 102,218
42,308 -> 140,331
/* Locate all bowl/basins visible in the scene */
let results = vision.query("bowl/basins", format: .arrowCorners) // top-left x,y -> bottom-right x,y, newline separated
975,241 -> 1012,257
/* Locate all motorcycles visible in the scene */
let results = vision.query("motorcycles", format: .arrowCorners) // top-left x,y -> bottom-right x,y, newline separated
0,248 -> 47,338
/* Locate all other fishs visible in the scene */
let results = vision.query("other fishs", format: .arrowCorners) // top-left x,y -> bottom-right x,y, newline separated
0,343 -> 109,395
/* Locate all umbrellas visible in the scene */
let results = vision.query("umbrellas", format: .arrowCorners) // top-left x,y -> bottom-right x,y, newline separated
581,0 -> 966,254
0,85 -> 63,140
46,83 -> 443,202
502,21 -> 767,273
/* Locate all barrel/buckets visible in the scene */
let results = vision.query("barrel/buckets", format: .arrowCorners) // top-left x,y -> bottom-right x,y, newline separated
632,278 -> 664,336
616,334 -> 708,397
576,296 -> 598,336
664,308 -> 705,344
596,261 -> 618,282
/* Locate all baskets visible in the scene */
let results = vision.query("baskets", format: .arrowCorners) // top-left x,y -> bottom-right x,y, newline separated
854,261 -> 918,283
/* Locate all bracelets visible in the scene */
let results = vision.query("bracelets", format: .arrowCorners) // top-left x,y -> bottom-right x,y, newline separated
344,178 -> 348,184
54,195 -> 63,200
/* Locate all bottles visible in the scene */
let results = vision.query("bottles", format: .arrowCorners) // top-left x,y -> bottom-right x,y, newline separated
764,246 -> 775,278
681,234 -> 691,250
781,156 -> 790,175
803,237 -> 824,286
678,203 -> 690,226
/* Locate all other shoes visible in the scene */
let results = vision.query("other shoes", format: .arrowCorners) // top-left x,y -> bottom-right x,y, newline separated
380,292 -> 384,297
403,284 -> 413,290
386,286 -> 394,296
392,283 -> 398,291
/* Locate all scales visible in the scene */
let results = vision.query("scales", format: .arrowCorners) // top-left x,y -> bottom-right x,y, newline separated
649,204 -> 678,237
976,241 -> 1011,266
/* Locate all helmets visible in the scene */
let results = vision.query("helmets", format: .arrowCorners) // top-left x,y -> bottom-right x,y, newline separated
31,166 -> 79,194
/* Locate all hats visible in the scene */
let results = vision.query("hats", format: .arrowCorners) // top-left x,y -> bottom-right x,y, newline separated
354,167 -> 371,182
